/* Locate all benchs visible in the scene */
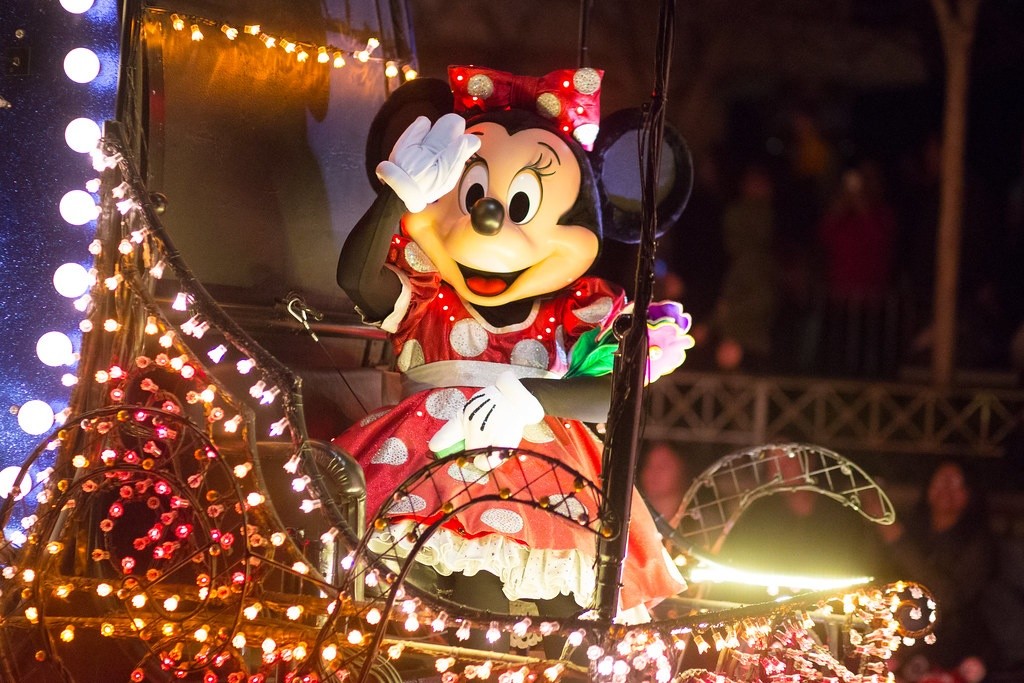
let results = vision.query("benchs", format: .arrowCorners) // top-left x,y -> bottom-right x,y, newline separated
138,305 -> 410,538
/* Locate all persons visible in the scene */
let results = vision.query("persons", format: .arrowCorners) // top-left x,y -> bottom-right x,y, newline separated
735,433 -> 870,575
335,65 -> 696,683
643,442 -> 702,544
856,466 -> 996,682
712,167 -> 780,364
815,162 -> 899,379
